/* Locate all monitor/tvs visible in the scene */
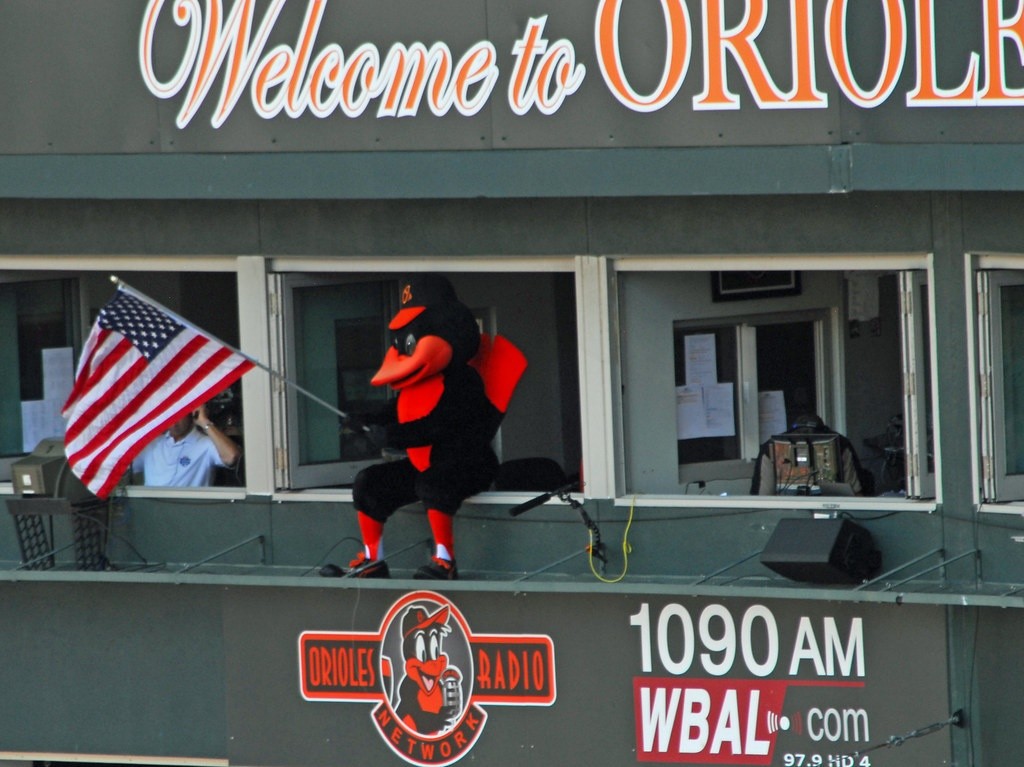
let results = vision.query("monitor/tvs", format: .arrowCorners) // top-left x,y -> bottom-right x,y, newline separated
10,437 -> 108,507
772,434 -> 844,495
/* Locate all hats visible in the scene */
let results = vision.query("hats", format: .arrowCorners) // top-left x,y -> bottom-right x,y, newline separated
388,273 -> 459,331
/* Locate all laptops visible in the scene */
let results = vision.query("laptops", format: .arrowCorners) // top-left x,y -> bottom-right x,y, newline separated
819,481 -> 857,498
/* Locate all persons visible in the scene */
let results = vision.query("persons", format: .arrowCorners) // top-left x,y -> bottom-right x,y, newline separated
120,404 -> 242,489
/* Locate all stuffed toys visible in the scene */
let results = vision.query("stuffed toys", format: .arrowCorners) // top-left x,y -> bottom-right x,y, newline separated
337,281 -> 525,576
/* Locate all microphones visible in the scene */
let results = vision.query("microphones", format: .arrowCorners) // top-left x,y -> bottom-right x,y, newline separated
506,484 -> 577,518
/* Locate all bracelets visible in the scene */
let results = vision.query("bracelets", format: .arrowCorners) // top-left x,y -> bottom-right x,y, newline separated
201,421 -> 214,430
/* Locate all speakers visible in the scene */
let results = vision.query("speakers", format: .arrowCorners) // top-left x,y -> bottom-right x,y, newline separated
759,516 -> 877,585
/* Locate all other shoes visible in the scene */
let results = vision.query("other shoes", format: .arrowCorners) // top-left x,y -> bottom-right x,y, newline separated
317,551 -> 391,577
412,555 -> 460,580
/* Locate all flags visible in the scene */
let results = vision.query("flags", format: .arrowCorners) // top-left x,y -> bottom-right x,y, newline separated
61,290 -> 255,501
